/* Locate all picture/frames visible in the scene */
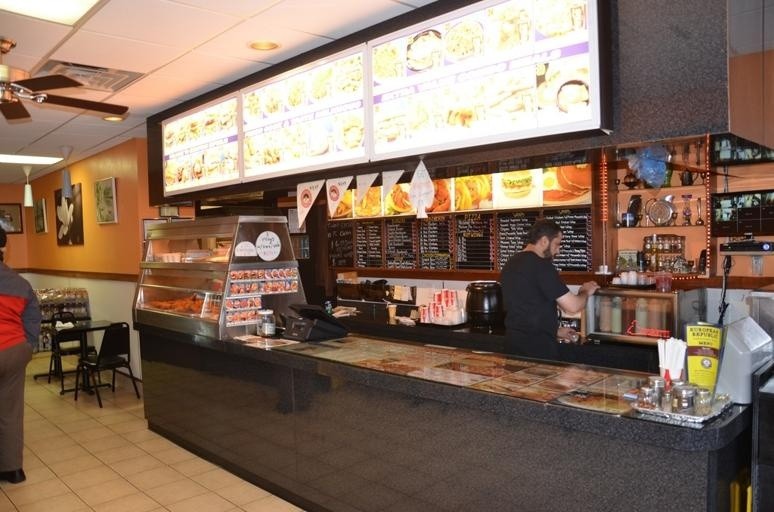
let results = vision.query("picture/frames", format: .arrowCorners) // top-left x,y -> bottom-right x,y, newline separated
93,177 -> 118,225
32,198 -> 47,236
0,202 -> 23,235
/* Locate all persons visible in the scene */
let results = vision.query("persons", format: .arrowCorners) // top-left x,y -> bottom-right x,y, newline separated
0,225 -> 42,484
498,219 -> 602,361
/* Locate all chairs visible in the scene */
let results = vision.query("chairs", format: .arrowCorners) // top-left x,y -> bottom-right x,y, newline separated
45,310 -> 143,408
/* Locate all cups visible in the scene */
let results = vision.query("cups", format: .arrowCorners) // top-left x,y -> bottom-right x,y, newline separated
419,290 -> 467,325
612,270 -> 672,292
387,305 -> 398,323
621,213 -> 638,228
660,367 -> 681,379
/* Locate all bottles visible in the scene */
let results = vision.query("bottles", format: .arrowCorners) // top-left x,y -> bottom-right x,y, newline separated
639,376 -> 712,417
256,309 -> 277,336
642,237 -> 682,253
598,298 -> 669,335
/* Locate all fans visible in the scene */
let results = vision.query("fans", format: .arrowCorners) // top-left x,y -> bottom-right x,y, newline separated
0,35 -> 130,126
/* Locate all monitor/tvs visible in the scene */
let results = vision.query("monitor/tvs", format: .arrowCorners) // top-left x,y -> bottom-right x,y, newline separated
289,304 -> 353,332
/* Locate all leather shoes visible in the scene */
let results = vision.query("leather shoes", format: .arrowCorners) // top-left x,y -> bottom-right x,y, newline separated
0,469 -> 26,483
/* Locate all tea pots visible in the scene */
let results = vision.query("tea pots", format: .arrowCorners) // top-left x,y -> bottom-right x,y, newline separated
678,168 -> 699,186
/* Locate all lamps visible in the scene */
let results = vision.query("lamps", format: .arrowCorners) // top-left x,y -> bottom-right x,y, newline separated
21,166 -> 35,208
57,144 -> 74,199
